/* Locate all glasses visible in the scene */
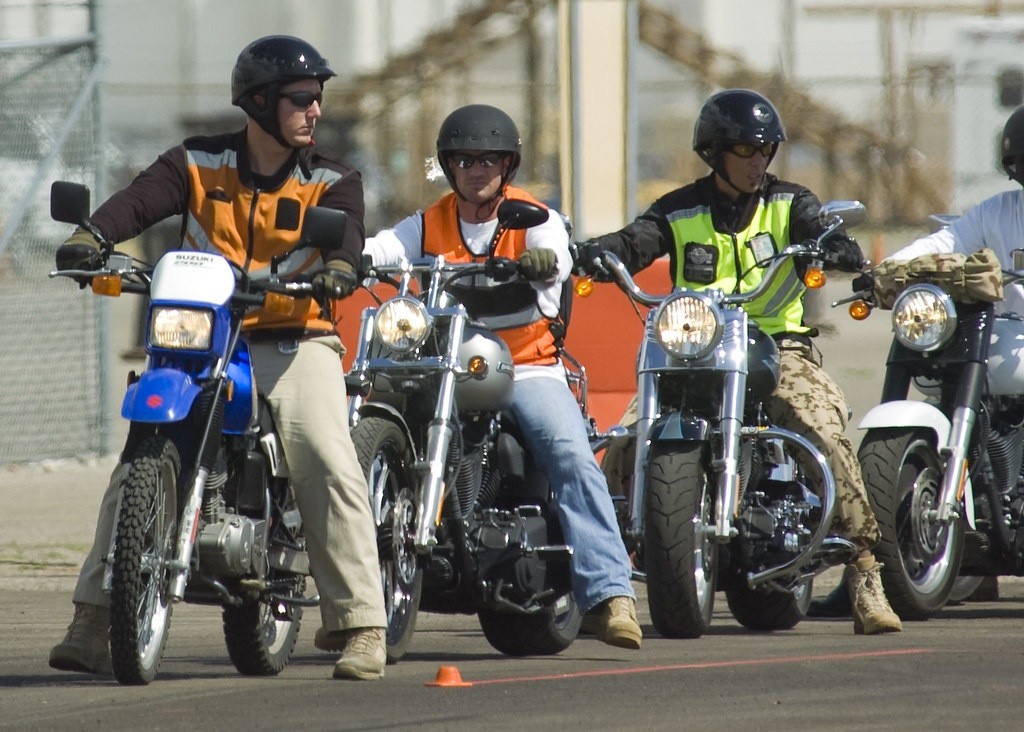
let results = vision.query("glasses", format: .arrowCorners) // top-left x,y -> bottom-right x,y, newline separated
279,90 -> 323,107
447,151 -> 506,169
722,143 -> 772,157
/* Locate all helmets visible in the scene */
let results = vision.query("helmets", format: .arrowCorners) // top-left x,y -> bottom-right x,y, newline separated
1001,104 -> 1024,185
692,89 -> 786,150
436,103 -> 522,190
230,35 -> 337,108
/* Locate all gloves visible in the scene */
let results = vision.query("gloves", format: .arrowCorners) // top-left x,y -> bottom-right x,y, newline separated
56,228 -> 103,290
830,236 -> 863,272
852,274 -> 875,304
569,239 -> 605,277
520,247 -> 556,281
312,259 -> 354,300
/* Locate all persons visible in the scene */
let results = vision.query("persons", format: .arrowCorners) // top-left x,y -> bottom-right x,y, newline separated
566,89 -> 902,636
50,34 -> 389,681
317,103 -> 642,651
859,107 -> 1024,603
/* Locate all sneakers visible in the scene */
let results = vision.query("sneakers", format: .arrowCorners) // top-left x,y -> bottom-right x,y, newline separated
580,605 -> 597,635
846,561 -> 903,634
332,626 -> 387,680
48,600 -> 113,676
314,626 -> 346,653
596,594 -> 643,650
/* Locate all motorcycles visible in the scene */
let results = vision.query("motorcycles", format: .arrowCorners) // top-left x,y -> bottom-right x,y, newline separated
571,199 -> 869,639
339,196 -> 589,670
830,211 -> 1024,620
49,186 -> 350,686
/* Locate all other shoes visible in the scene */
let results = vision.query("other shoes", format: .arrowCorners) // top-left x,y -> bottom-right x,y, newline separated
968,575 -> 998,599
